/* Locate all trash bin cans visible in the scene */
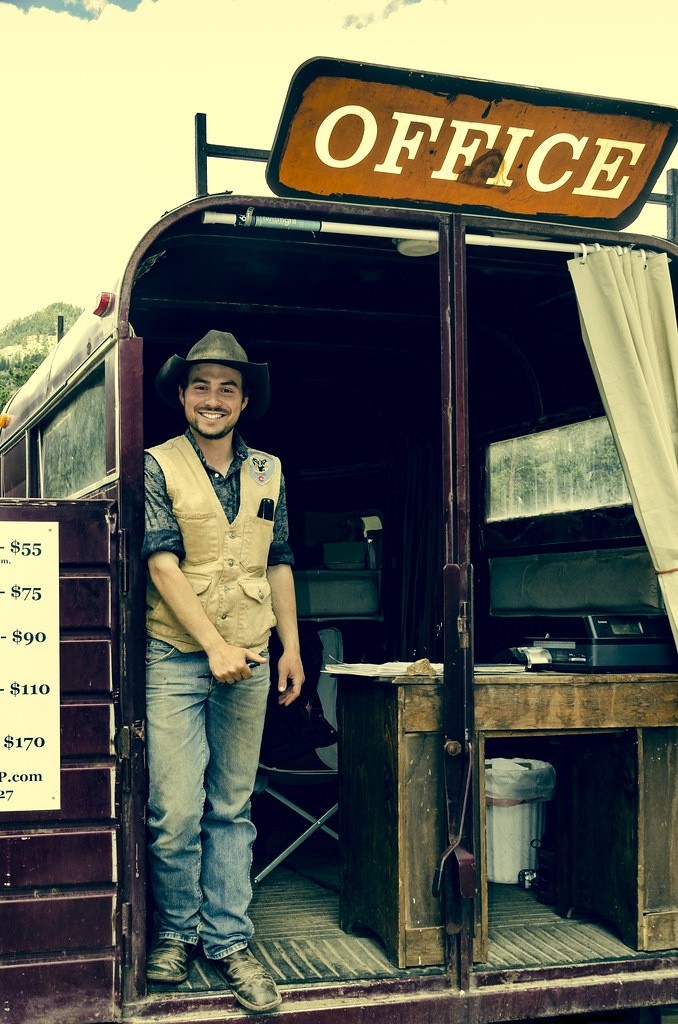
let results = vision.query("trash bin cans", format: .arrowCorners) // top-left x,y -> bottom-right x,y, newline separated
484,759 -> 555,885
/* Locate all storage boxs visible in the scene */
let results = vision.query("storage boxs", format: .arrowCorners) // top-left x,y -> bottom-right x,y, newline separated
287,568 -> 379,616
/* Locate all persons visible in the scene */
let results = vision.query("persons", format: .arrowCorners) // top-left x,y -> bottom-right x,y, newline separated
145,331 -> 306,1011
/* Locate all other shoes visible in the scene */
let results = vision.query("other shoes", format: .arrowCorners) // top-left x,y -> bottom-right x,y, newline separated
211,949 -> 282,1012
146,939 -> 201,982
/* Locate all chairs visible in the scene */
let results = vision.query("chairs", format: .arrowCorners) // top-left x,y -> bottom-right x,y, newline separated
253,619 -> 339,886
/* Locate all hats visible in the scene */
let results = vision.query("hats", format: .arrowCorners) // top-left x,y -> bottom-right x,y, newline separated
155,330 -> 270,422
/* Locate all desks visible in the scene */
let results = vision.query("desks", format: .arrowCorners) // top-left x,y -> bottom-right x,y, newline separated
328,674 -> 678,971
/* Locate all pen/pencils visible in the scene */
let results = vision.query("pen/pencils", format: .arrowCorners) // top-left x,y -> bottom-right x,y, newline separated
197,661 -> 262,679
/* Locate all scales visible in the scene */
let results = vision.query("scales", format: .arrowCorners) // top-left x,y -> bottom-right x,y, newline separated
524,615 -> 678,673
